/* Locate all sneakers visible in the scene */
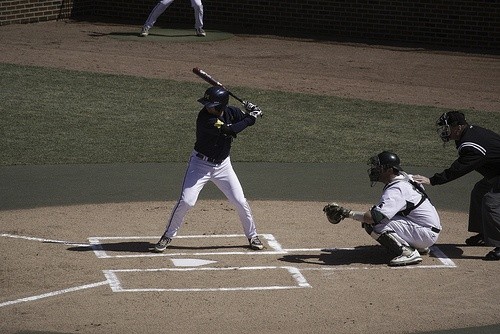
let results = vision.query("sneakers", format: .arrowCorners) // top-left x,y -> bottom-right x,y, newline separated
483,248 -> 500,261
140,27 -> 149,36
155,236 -> 171,252
390,248 -> 430,264
196,28 -> 205,36
465,234 -> 488,245
248,237 -> 265,250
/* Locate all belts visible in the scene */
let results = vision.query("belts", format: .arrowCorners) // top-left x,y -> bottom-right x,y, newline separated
196,153 -> 226,164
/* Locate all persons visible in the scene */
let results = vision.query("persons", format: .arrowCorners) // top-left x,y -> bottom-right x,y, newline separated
154,87 -> 264,250
140,0 -> 206,38
412,111 -> 500,261
322,152 -> 442,266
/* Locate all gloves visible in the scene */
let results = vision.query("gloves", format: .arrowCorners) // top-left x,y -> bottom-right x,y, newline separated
242,100 -> 263,120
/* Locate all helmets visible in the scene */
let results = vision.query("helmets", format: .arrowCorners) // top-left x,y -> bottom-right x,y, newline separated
372,151 -> 405,172
197,86 -> 229,112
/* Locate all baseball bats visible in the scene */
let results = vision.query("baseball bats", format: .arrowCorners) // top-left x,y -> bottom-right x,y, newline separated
193,67 -> 246,105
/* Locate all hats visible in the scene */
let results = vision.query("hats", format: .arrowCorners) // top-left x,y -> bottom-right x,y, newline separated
438,111 -> 469,125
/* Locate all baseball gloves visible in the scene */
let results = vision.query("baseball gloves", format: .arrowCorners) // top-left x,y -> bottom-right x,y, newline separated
323,203 -> 351,224
